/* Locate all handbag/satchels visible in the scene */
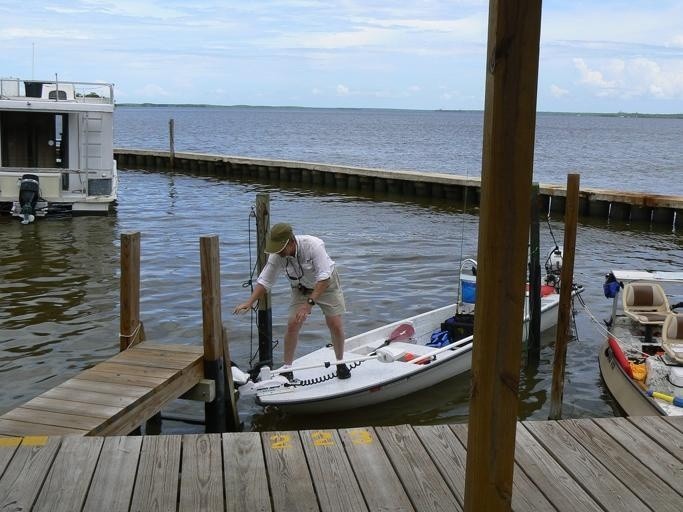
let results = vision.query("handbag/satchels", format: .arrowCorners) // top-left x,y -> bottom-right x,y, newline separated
445,313 -> 475,349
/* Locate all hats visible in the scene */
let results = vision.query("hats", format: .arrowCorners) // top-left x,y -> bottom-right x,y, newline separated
263,223 -> 292,254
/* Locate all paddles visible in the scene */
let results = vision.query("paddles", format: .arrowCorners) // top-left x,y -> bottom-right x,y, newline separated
369,324 -> 415,356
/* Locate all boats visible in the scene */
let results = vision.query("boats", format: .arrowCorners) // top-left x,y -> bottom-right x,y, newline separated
0,73 -> 117,217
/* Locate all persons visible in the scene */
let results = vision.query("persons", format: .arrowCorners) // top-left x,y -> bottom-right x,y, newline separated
232,222 -> 353,382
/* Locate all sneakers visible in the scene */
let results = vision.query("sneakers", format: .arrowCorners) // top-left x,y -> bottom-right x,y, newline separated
336,364 -> 351,379
279,371 -> 294,382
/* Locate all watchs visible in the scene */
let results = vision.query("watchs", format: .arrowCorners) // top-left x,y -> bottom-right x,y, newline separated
307,298 -> 316,306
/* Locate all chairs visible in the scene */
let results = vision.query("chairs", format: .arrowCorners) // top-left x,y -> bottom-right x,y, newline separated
620,280 -> 683,368
